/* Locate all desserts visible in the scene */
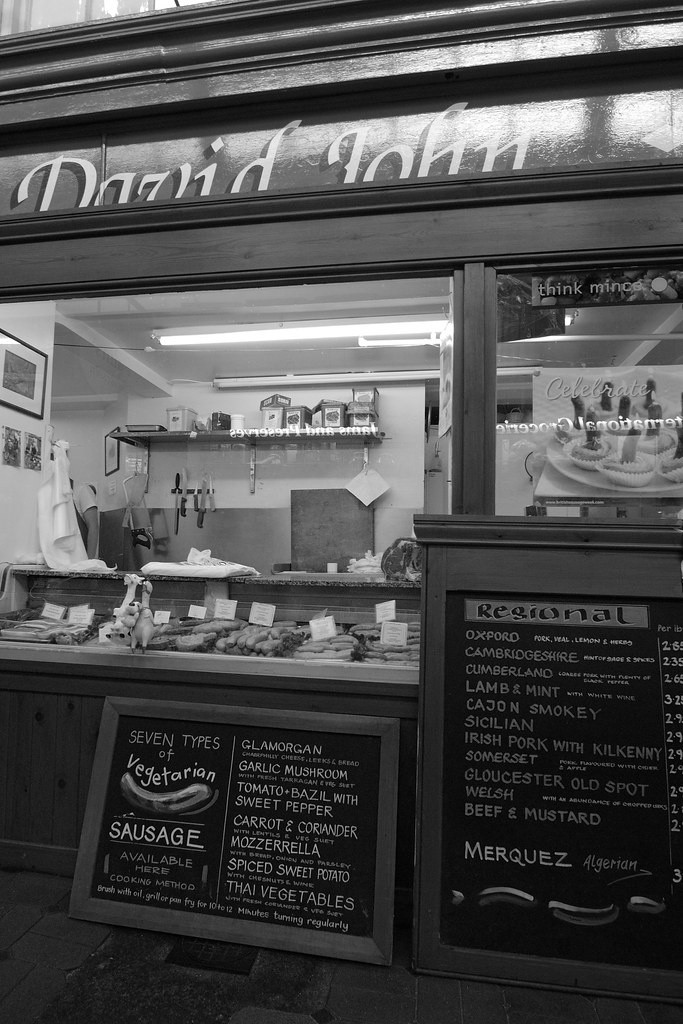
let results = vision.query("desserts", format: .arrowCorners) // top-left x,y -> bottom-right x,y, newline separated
559,400 -> 683,474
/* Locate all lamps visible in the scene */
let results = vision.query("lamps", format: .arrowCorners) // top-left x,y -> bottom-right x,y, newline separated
153,309 -> 579,348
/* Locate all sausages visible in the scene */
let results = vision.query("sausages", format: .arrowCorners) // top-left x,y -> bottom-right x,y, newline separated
121,773 -> 211,814
193,619 -> 420,666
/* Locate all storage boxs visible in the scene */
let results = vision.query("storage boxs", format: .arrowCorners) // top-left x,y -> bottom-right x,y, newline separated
259,385 -> 380,427
165,405 -> 197,434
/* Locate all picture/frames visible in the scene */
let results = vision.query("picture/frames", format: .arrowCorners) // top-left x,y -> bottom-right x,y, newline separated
0,327 -> 48,419
67,694 -> 403,969
104,426 -> 120,477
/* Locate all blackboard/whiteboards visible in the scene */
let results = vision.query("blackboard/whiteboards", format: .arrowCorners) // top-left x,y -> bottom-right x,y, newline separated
439,588 -> 683,973
64,694 -> 400,968
